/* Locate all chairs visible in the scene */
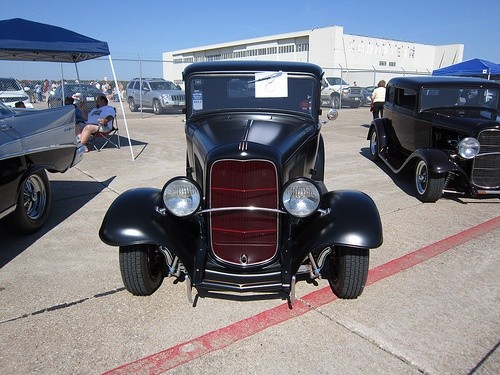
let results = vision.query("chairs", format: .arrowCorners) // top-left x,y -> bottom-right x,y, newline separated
5,82 -> 15,91
400,90 -> 486,116
206,85 -> 309,116
77,106 -> 121,152
136,83 -> 171,92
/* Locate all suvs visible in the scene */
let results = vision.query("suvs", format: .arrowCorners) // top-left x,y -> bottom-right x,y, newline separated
126,77 -> 186,115
320,76 -> 364,109
0,76 -> 30,109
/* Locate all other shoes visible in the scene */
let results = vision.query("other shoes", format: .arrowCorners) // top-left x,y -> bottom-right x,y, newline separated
84,146 -> 89,152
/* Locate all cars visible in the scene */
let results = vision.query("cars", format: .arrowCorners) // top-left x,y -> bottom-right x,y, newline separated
364,75 -> 500,204
359,86 -> 378,107
47,82 -> 106,113
98,59 -> 384,311
0,97 -> 86,235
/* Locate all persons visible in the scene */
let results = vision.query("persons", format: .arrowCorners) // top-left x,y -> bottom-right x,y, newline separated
21,79 -> 124,105
64,93 -> 88,121
76,94 -> 116,153
372,80 -> 387,119
15,101 -> 26,108
